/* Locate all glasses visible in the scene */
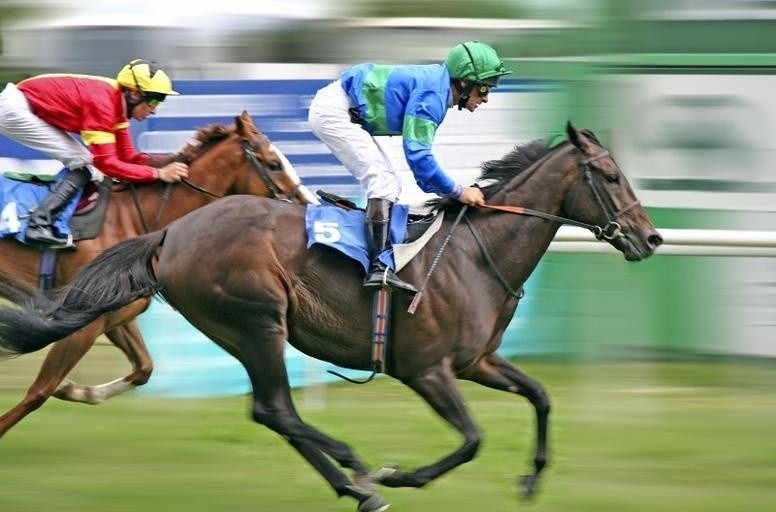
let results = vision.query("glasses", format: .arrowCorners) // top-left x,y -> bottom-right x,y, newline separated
477,84 -> 490,97
145,98 -> 160,109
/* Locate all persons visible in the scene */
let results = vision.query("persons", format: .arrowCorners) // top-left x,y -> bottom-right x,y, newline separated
307,39 -> 513,300
0,56 -> 190,251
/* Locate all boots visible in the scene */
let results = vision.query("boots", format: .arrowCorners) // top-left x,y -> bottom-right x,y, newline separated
25,179 -> 79,251
362,198 -> 418,296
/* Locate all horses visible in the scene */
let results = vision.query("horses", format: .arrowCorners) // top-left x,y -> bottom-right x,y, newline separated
0,109 -> 321,438
0,118 -> 664,512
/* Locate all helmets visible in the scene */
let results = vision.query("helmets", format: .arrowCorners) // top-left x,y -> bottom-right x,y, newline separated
445,41 -> 514,87
117,59 -> 179,95
